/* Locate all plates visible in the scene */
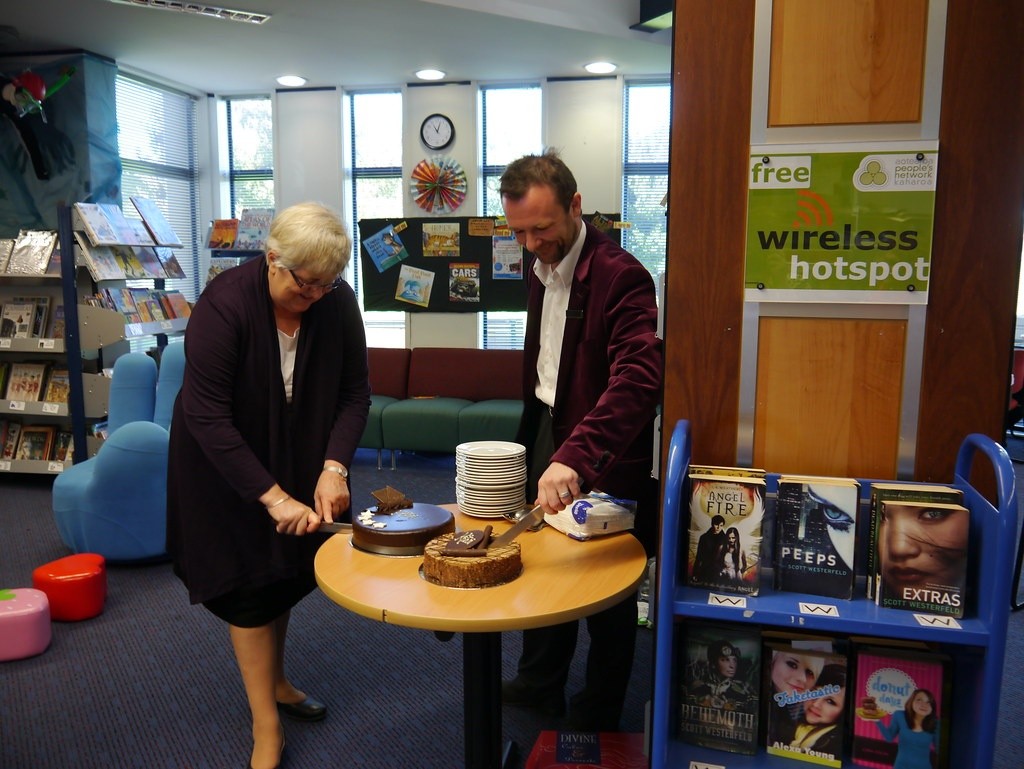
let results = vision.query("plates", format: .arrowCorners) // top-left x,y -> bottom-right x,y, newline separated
454,440 -> 527,518
855,706 -> 890,720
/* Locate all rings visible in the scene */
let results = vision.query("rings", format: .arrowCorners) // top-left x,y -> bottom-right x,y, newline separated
560,491 -> 569,498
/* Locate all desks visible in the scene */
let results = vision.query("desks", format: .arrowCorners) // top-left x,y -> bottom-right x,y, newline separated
314,503 -> 648,769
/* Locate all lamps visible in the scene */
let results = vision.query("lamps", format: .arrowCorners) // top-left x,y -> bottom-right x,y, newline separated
628,0 -> 672,33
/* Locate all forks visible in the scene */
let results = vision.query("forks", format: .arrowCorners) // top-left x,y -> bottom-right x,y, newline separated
524,522 -> 549,532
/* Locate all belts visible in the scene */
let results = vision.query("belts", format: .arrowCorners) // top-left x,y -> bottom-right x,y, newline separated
540,401 -> 555,418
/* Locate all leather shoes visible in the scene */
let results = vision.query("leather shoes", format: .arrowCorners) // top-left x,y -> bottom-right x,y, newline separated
502,672 -> 566,718
276,690 -> 328,722
247,725 -> 286,769
575,701 -> 618,732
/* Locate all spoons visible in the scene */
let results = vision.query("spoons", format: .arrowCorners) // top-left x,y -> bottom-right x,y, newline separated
502,510 -> 533,523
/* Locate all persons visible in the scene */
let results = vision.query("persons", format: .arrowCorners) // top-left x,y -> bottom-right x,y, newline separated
875,688 -> 940,769
789,664 -> 846,760
499,155 -> 663,733
692,515 -> 726,581
769,650 -> 824,747
694,639 -> 746,701
807,484 -> 857,570
878,504 -> 969,613
714,527 -> 747,585
165,203 -> 372,769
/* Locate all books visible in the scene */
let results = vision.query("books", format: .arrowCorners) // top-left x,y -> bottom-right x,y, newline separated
205,207 -> 276,286
685,464 -> 969,618
677,626 -> 943,769
0,229 -> 74,461
73,196 -> 191,323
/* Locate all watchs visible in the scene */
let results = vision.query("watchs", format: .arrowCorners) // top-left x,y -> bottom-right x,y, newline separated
324,466 -> 347,477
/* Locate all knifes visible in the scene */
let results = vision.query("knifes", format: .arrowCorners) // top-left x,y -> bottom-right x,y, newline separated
271,517 -> 354,534
486,505 -> 545,548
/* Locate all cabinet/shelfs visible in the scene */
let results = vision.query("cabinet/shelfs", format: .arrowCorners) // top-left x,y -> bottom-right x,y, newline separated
0,205 -> 189,491
650,419 -> 1020,769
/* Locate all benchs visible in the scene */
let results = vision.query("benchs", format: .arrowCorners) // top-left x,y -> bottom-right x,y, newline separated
357,347 -> 412,471
381,347 -> 523,472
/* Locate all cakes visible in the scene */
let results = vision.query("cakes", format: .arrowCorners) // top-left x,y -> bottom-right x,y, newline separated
423,525 -> 521,588
351,485 -> 455,556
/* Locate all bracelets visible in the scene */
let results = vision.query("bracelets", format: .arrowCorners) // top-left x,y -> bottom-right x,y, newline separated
265,495 -> 289,509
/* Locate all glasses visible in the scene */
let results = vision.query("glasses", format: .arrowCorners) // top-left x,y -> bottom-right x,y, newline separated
290,270 -> 342,294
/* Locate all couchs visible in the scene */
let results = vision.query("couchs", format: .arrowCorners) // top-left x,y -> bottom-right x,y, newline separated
51,339 -> 185,568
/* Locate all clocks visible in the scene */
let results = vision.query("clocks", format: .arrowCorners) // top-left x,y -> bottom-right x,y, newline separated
420,113 -> 455,150
